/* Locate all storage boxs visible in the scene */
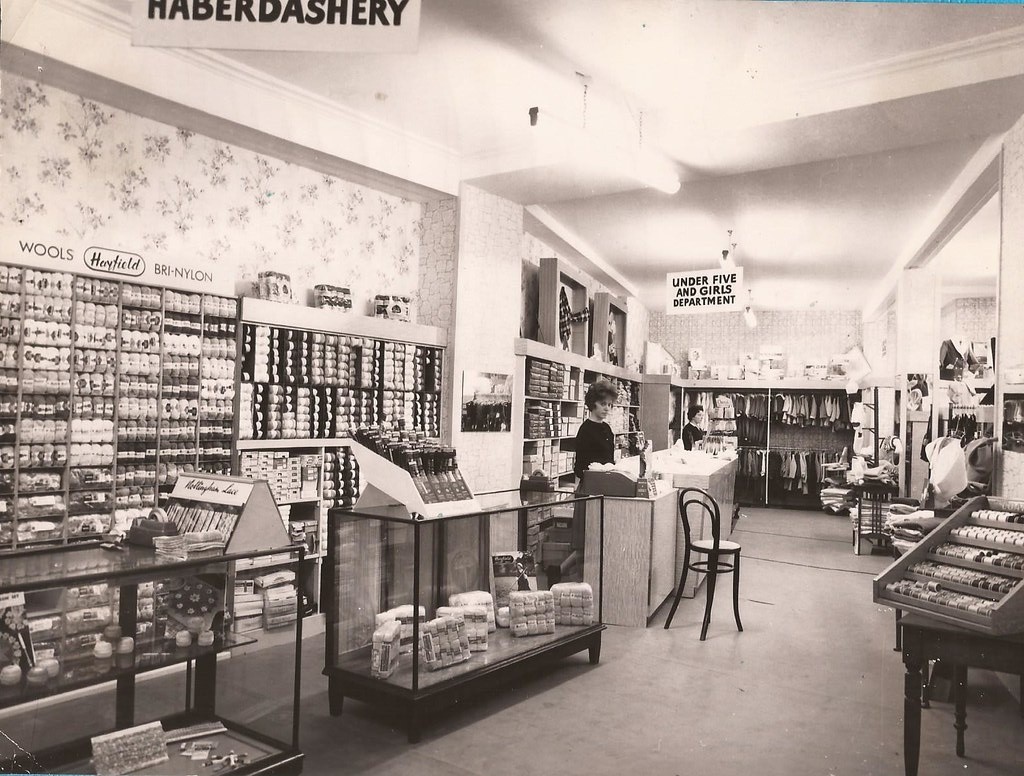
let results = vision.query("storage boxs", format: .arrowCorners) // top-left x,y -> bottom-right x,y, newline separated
524,442 -> 576,568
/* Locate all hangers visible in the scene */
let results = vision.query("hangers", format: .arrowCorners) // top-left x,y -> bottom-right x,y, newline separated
771,446 -> 836,455
952,405 -> 977,416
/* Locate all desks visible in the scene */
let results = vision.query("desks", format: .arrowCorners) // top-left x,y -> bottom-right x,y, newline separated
899,613 -> 1024,776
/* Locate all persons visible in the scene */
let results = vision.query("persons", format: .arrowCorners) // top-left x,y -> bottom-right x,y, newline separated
682,406 -> 704,450
545,381 -> 616,593
940,339 -> 980,381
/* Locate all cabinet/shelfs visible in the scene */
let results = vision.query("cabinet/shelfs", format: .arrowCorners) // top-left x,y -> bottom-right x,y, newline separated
852,379 -> 898,558
515,259 -> 643,570
641,376 -> 861,513
1,536 -> 305,775
0,259 -> 449,661
323,490 -> 607,744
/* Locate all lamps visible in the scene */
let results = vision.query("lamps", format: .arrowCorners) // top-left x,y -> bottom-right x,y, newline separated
530,72 -> 681,195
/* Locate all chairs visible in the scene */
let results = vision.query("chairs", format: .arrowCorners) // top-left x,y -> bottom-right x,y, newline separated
664,487 -> 743,640
